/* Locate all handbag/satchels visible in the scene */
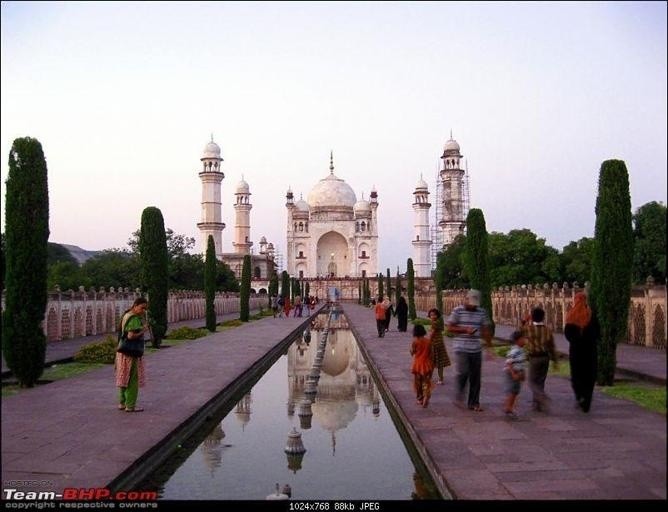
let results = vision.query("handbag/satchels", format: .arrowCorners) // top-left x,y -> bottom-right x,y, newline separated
117,331 -> 144,356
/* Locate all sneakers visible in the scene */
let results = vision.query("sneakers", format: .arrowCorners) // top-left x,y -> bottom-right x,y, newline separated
119,404 -> 143,412
416,381 -> 444,408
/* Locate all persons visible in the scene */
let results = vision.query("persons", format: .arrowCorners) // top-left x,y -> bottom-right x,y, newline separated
369,295 -> 408,338
409,325 -> 434,408
303,328 -> 311,346
518,308 -> 558,412
447,288 -> 492,412
563,291 -> 601,413
427,309 -> 451,385
297,337 -> 303,349
271,293 -> 318,318
114,297 -> 150,411
500,330 -> 526,420
